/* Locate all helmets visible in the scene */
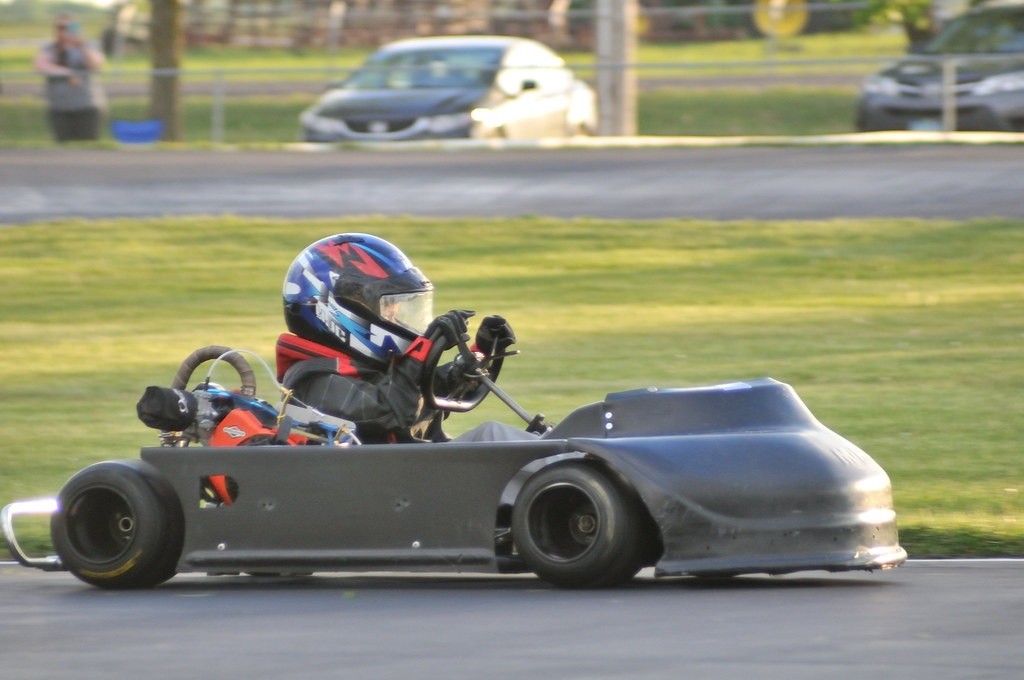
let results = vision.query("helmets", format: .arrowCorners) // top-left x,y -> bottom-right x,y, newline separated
282,232 -> 435,369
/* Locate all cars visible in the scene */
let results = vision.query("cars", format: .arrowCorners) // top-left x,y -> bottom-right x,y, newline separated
301,30 -> 600,145
843,3 -> 1024,135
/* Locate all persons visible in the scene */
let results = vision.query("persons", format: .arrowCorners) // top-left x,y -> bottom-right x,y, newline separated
275,233 -> 540,442
37,15 -> 105,141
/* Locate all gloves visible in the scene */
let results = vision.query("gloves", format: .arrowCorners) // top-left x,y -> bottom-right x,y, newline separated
413,309 -> 476,364
477,314 -> 517,360
